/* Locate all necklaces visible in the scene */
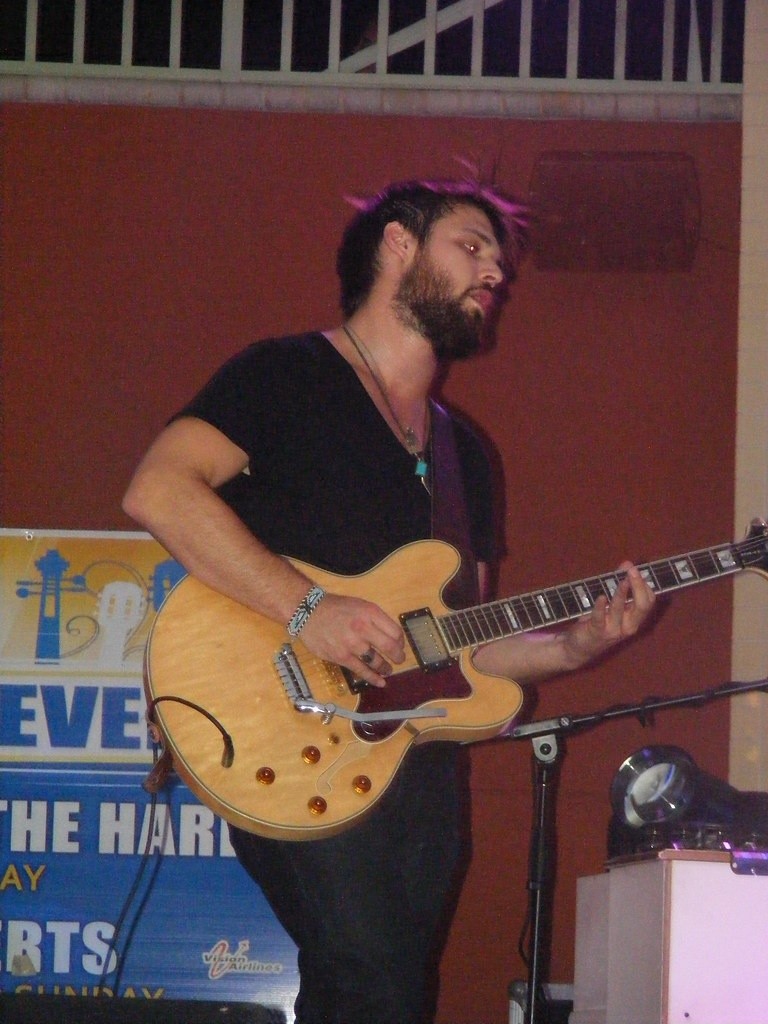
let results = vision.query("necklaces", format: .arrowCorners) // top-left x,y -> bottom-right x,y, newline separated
339,322 -> 434,501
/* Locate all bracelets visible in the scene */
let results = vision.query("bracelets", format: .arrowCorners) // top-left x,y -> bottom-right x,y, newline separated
286,584 -> 328,637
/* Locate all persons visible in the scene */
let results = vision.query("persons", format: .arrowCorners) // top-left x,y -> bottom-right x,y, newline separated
120,174 -> 661,1023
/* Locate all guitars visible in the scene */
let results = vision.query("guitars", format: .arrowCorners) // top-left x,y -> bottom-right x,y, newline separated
140,512 -> 767,841
